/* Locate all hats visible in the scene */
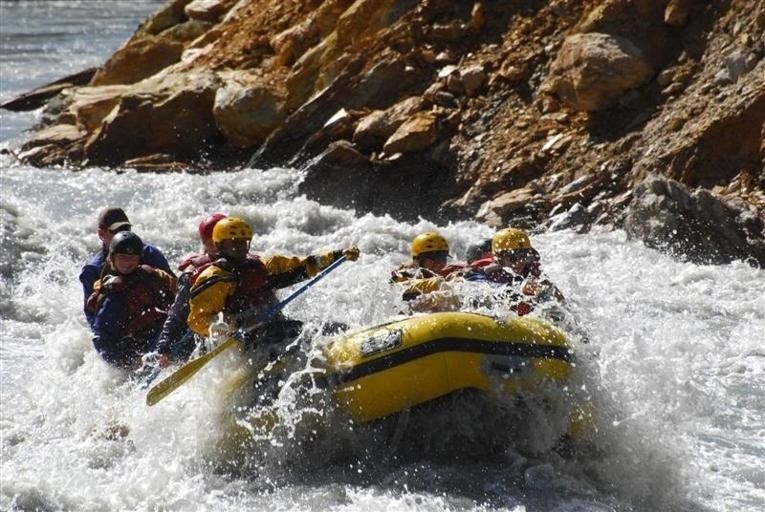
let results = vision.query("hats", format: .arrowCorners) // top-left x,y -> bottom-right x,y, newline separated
97,205 -> 133,231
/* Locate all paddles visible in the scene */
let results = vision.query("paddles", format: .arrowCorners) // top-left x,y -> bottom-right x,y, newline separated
146,246 -> 358,407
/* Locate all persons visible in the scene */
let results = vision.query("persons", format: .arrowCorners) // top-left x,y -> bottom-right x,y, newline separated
387,225 -> 591,346
185,217 -> 361,370
79,208 -> 177,329
154,213 -> 263,371
90,231 -> 178,373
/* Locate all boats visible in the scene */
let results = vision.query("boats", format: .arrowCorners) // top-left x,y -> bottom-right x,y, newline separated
218,308 -> 598,463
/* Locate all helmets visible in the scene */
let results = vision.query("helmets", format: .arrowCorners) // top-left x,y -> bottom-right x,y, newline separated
466,238 -> 492,261
491,227 -> 531,256
199,214 -> 227,241
411,231 -> 454,260
212,217 -> 253,243
110,231 -> 144,255
